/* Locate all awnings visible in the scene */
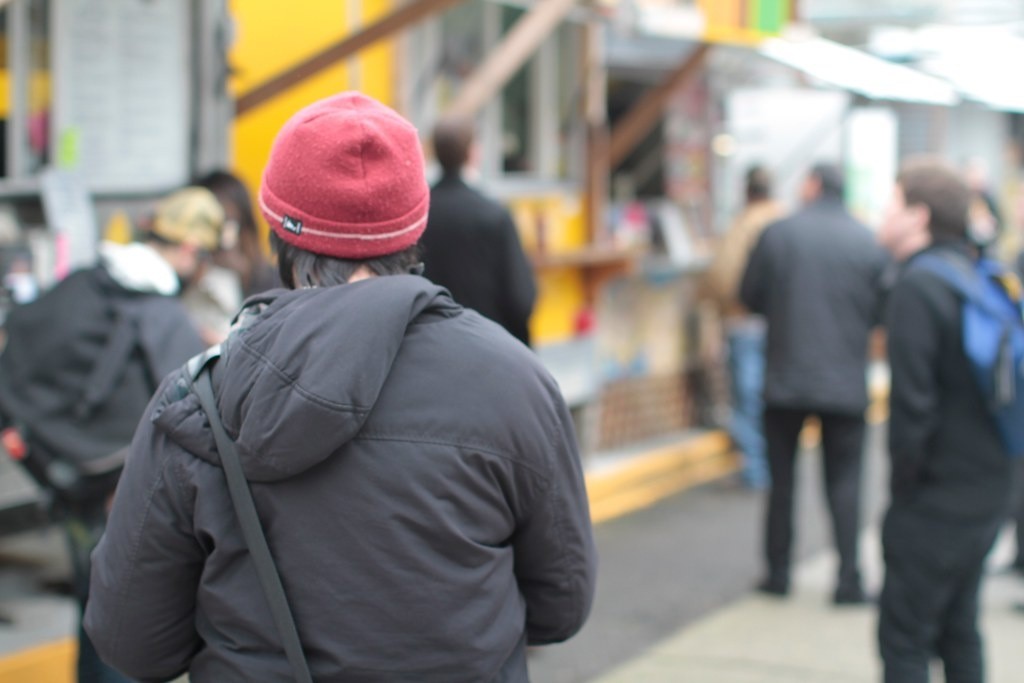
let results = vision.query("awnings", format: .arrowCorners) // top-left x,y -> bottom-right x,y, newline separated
863,22 -> 1023,117
238,2 -> 958,243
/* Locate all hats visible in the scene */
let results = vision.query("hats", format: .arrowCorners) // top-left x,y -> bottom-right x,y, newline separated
151,184 -> 225,250
260,91 -> 431,257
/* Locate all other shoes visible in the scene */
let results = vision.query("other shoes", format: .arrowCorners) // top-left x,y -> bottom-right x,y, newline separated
756,578 -> 788,595
834,586 -> 865,603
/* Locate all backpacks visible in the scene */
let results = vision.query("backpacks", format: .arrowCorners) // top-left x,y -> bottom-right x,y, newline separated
914,246 -> 1024,451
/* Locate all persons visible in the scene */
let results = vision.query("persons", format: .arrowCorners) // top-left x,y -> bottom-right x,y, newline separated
701,166 -> 790,498
86,94 -> 598,683
5,181 -> 224,516
876,158 -> 1012,683
418,122 -> 539,342
169,172 -> 262,354
743,164 -> 892,604
